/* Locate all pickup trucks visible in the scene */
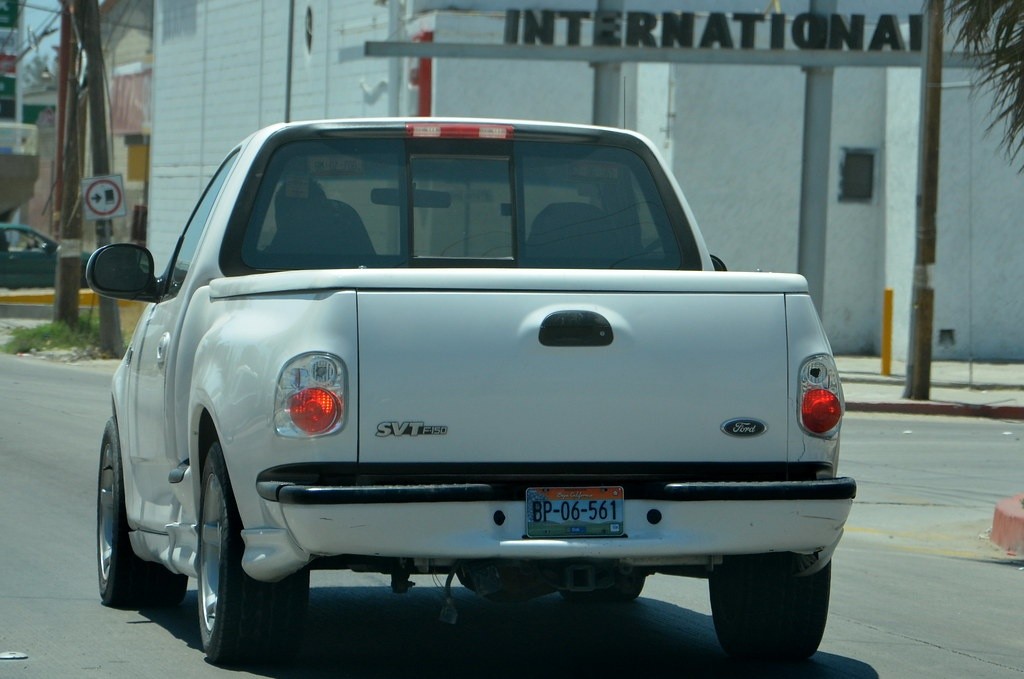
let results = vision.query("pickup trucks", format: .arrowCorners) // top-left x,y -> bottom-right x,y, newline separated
85,115 -> 858,670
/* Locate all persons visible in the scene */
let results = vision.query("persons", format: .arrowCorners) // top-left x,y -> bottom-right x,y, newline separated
260,179 -> 327,255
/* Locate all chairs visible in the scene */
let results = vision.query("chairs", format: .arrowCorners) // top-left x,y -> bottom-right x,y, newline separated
523,201 -> 631,269
265,199 -> 380,268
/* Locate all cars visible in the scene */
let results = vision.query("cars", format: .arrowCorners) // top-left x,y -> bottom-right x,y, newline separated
0,222 -> 93,291
